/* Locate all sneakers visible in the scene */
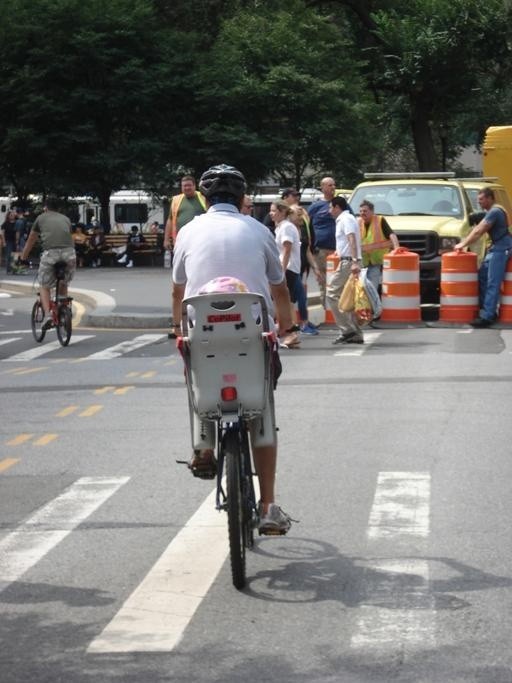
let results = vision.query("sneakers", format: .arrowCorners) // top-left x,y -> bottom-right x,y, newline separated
40,312 -> 53,331
257,500 -> 294,532
468,319 -> 490,328
189,441 -> 218,481
300,325 -> 320,336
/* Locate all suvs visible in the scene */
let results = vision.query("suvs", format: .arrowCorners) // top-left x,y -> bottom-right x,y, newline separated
325,168 -> 511,318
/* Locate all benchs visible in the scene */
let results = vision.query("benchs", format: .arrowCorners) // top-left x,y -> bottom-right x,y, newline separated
81,233 -> 161,268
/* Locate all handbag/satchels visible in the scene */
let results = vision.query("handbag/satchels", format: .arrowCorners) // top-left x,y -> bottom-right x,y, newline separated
336,269 -> 355,314
353,276 -> 375,328
358,274 -> 384,320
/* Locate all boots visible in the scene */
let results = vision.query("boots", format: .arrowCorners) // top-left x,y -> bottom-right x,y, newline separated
117,254 -> 128,264
126,259 -> 134,268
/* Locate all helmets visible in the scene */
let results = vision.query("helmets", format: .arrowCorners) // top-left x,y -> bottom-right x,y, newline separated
198,164 -> 248,198
198,275 -> 248,294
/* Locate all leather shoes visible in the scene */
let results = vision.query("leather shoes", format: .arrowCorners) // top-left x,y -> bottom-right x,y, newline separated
330,331 -> 356,344
347,338 -> 364,345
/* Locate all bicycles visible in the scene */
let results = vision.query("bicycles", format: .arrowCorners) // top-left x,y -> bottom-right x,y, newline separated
16,256 -> 77,348
163,292 -> 299,592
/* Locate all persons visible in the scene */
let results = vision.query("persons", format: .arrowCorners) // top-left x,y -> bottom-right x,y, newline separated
169,164 -> 293,535
193,277 -> 251,402
163,176 -> 206,252
0,205 -> 158,268
21,196 -> 77,331
455,188 -> 512,328
262,176 -> 400,345
239,195 -> 254,215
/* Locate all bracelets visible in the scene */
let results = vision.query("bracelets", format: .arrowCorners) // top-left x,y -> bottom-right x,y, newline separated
170,322 -> 181,328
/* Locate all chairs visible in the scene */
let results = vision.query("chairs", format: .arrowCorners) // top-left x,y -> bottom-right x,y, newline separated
178,291 -> 271,423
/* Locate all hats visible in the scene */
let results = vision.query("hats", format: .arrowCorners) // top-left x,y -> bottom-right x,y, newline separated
281,187 -> 303,196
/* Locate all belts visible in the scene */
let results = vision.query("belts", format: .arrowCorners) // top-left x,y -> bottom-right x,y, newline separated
339,256 -> 363,262
